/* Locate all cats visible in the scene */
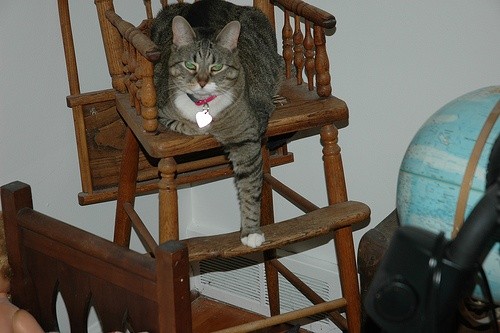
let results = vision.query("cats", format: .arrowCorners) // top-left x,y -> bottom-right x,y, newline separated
149,0 -> 298,249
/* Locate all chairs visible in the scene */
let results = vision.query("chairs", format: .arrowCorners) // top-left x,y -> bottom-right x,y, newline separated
94,0 -> 370,333
1,178 -> 194,333
